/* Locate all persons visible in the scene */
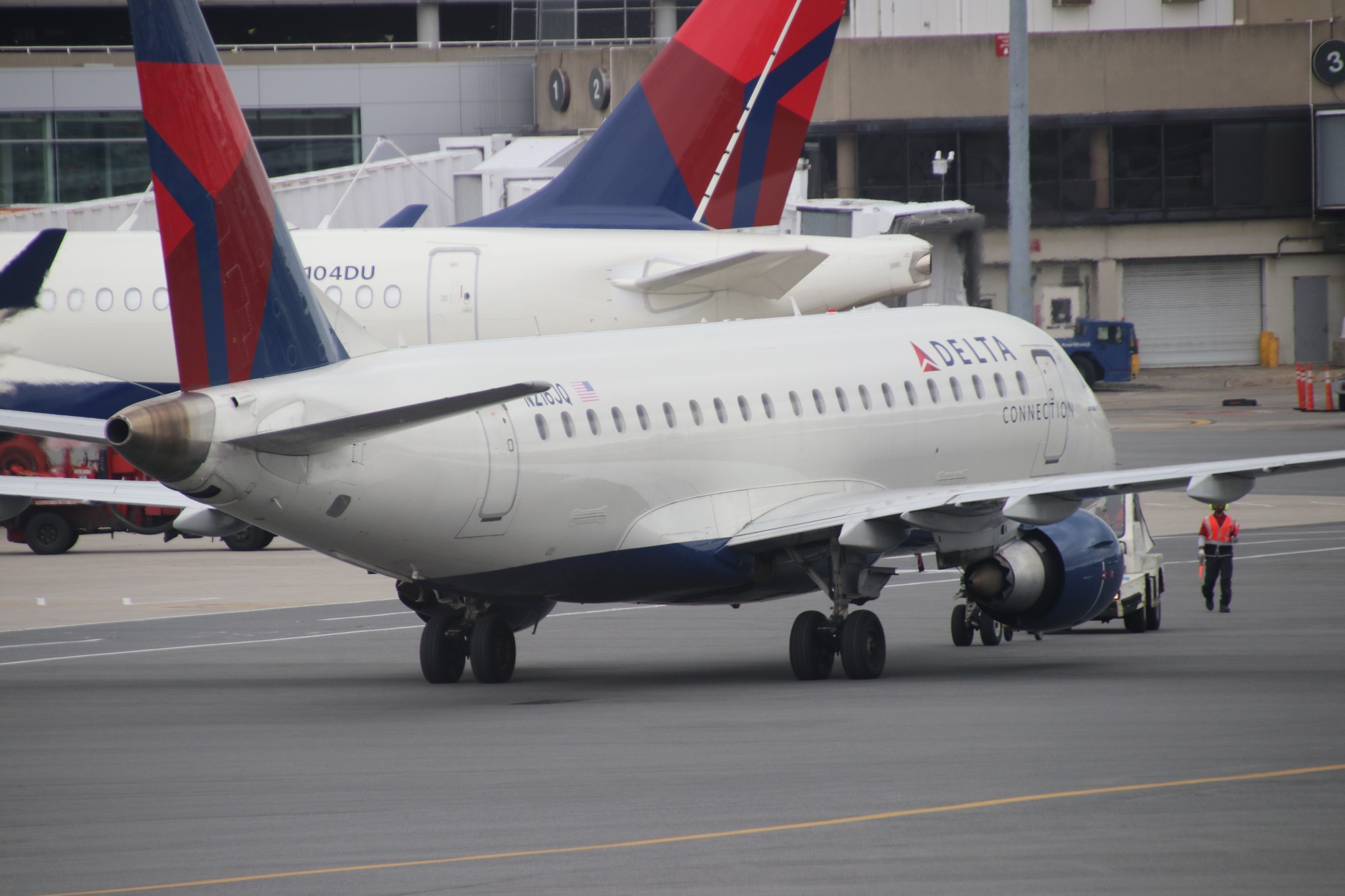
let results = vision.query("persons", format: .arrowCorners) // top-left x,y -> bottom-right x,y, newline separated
1199,503 -> 1240,612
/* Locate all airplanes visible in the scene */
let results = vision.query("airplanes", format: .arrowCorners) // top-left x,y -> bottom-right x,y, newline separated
0,0 -> 1345,682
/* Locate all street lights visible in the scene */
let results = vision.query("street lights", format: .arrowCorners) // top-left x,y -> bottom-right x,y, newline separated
934,150 -> 955,202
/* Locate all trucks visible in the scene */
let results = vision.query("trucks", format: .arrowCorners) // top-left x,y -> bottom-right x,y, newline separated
1052,317 -> 1141,391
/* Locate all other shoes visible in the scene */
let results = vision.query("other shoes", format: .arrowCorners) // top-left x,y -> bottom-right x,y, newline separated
1219,599 -> 1230,613
1205,597 -> 1215,610
